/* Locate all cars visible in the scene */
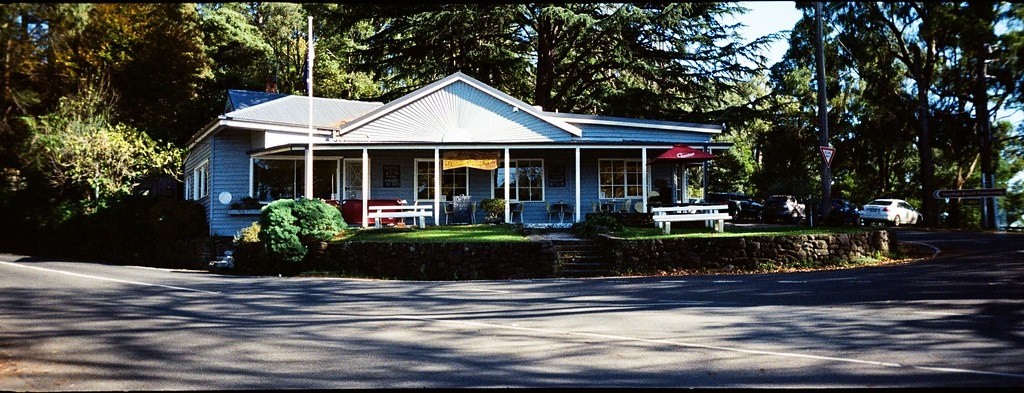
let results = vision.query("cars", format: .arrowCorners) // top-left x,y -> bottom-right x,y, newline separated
859,198 -> 925,227
816,198 -> 862,225
706,192 -> 764,223
763,195 -> 805,225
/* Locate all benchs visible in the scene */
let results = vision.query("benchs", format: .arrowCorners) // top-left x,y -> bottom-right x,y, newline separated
652,213 -> 732,234
368,211 -> 433,229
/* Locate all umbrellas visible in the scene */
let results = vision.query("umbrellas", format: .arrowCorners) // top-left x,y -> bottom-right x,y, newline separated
647,144 -> 721,167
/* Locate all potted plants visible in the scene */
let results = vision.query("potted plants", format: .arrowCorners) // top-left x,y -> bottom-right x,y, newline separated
479,199 -> 506,226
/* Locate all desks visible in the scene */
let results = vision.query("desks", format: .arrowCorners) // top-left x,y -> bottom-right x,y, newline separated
652,204 -> 728,229
552,202 -> 568,222
369,204 -> 433,210
604,202 -> 624,213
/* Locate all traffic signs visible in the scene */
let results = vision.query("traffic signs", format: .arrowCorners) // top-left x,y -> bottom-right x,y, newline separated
934,188 -> 1007,199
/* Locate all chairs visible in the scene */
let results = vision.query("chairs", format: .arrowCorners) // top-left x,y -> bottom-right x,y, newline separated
510,202 -> 524,224
598,199 -> 613,214
561,205 -> 576,223
444,201 -> 453,226
618,199 -> 632,212
546,200 -> 562,223
470,201 -> 477,225
